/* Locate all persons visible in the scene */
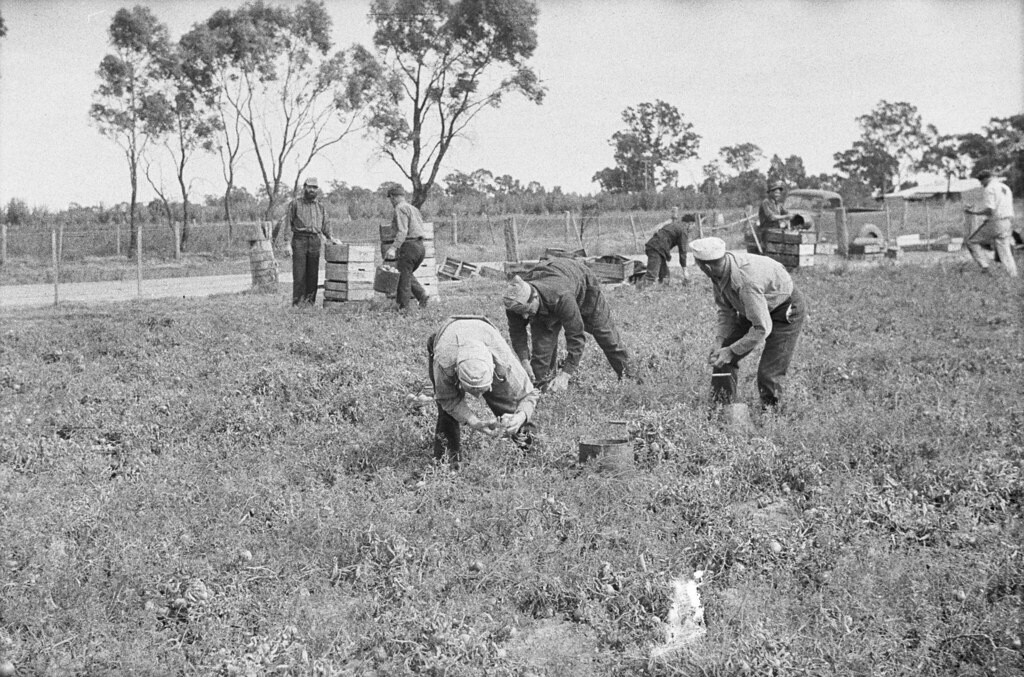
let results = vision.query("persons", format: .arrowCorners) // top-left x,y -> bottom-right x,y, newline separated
383,186 -> 429,312
758,185 -> 795,231
690,239 -> 804,421
502,257 -> 631,392
962,169 -> 1020,278
426,314 -> 539,462
640,213 -> 695,284
284,176 -> 344,315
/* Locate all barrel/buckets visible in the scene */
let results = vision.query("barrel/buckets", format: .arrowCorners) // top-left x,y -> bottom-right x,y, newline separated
709,374 -> 752,428
790,214 -> 811,230
372,257 -> 400,293
578,420 -> 635,468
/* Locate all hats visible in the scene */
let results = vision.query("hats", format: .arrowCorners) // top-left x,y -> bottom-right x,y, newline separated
386,187 -> 405,198
504,275 -> 532,311
689,237 -> 726,261
766,183 -> 782,193
456,350 -> 493,392
304,176 -> 319,187
977,169 -> 991,180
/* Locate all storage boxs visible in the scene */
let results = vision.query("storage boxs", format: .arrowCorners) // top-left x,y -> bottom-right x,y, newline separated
585,255 -> 634,279
766,229 -> 817,268
373,223 -> 438,297
849,244 -> 879,255
323,243 -> 376,311
